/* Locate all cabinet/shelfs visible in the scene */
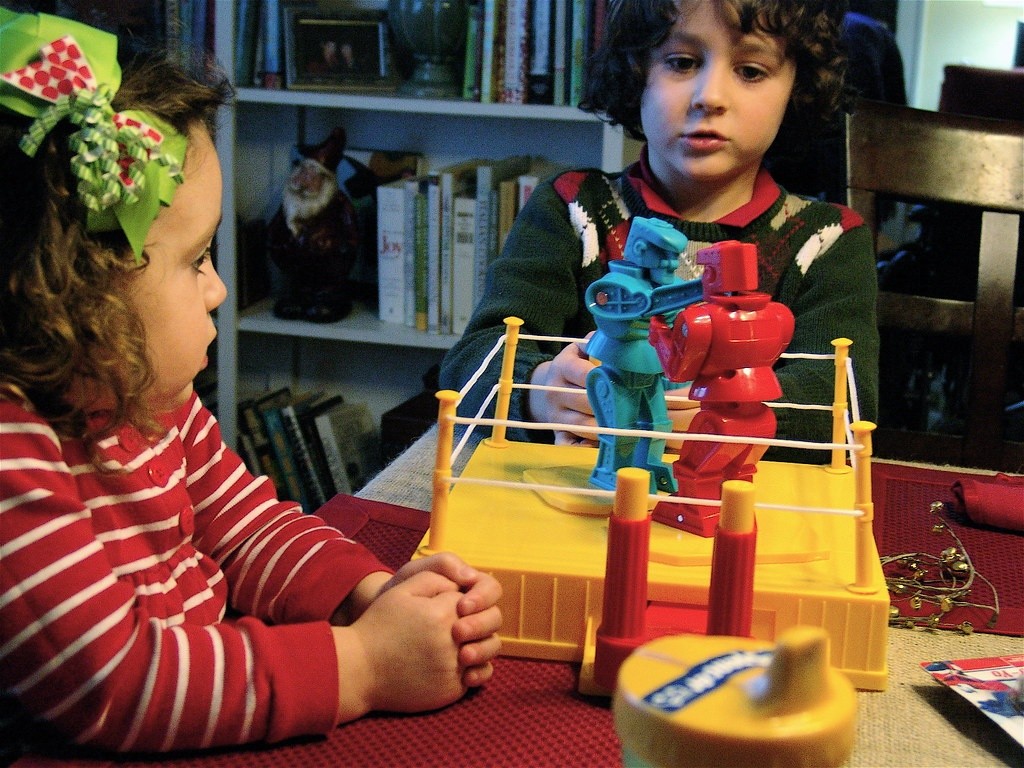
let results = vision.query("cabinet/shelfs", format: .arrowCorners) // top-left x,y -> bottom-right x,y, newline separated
214,0 -> 624,516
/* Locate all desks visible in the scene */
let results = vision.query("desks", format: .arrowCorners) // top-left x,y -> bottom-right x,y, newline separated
5,420 -> 1024,768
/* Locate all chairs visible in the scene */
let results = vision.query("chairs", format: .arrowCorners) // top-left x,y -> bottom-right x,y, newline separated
845,96 -> 1024,474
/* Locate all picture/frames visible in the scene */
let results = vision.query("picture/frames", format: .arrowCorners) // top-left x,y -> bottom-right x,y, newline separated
284,6 -> 396,93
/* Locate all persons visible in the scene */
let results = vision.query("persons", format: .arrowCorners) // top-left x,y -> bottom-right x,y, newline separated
0,15 -> 501,750
831,0 -> 906,221
584,217 -> 704,493
306,39 -> 368,85
440,0 -> 880,460
650,241 -> 794,537
268,125 -> 356,324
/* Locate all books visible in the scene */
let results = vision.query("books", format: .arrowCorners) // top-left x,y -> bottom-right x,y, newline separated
460,0 -> 610,104
237,387 -> 382,511
235,0 -> 282,90
166,0 -> 211,76
377,156 -> 560,336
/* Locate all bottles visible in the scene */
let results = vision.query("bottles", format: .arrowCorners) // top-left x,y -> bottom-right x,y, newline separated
612,623 -> 859,768
